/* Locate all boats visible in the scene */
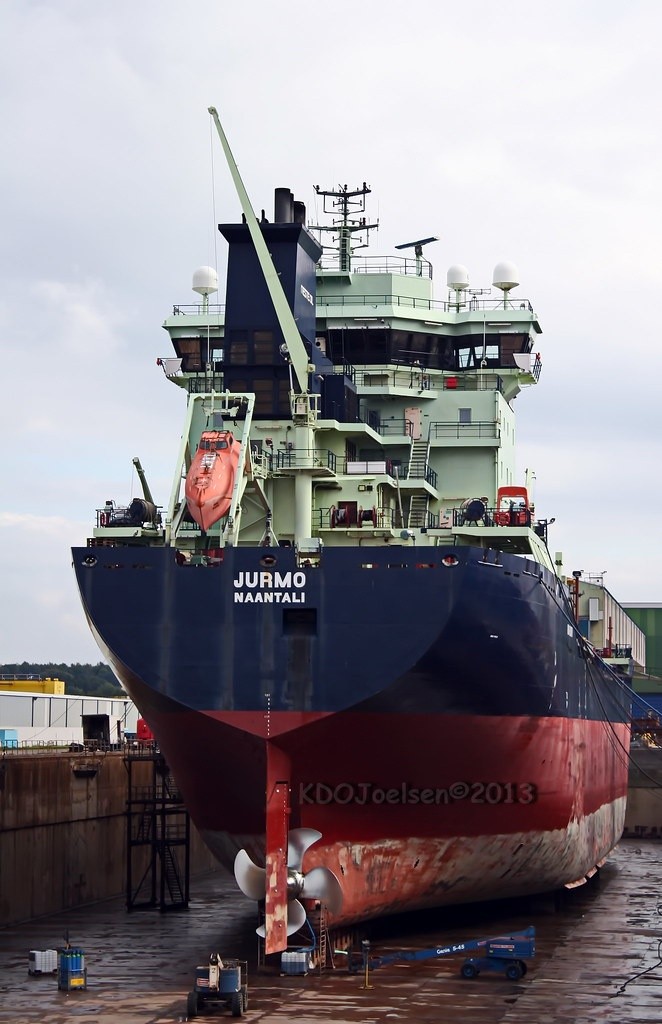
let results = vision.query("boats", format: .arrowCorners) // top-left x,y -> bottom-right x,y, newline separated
69,109 -> 645,961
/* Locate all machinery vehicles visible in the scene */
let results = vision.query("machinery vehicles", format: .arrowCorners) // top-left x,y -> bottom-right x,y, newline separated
185,952 -> 248,1020
